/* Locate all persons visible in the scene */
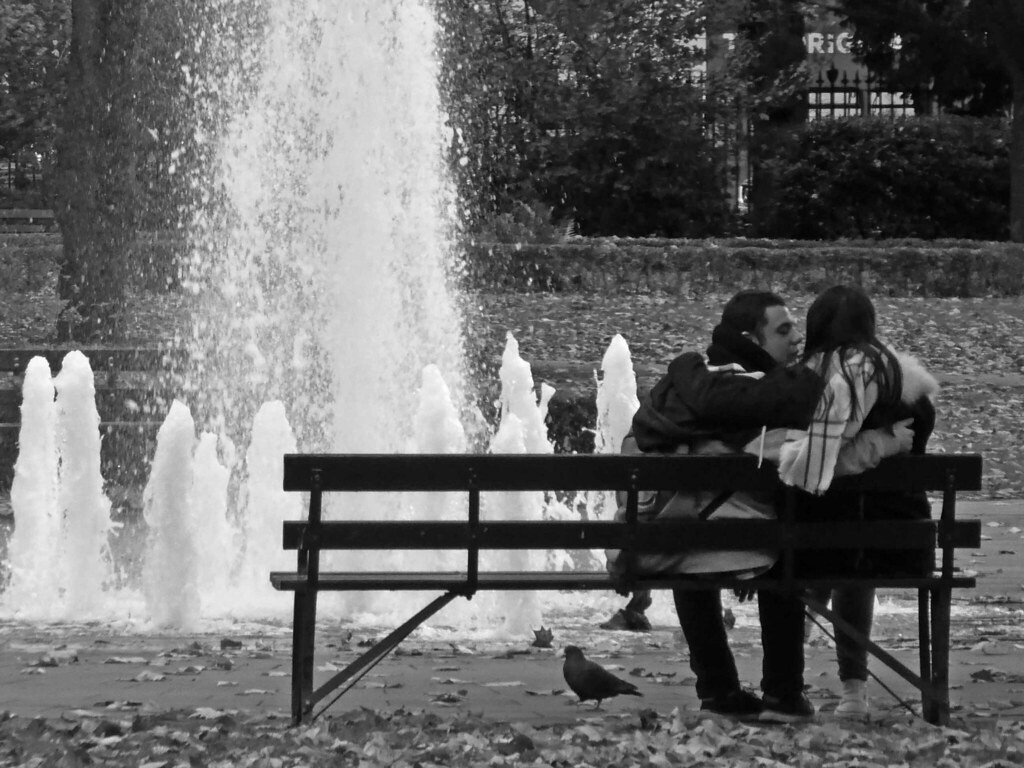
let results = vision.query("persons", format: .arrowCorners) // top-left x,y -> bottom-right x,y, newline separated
614,290 -> 913,722
713,285 -> 942,718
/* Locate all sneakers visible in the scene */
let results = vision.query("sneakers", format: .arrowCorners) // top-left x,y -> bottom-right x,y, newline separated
833,686 -> 868,718
699,687 -> 763,725
759,685 -> 818,724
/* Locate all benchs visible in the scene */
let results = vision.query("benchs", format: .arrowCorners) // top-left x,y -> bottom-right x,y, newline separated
270,453 -> 982,732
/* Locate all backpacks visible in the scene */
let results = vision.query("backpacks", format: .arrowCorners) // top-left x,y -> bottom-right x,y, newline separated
604,425 -> 697,575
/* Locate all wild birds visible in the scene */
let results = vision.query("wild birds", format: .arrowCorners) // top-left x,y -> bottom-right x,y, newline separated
554,646 -> 644,712
